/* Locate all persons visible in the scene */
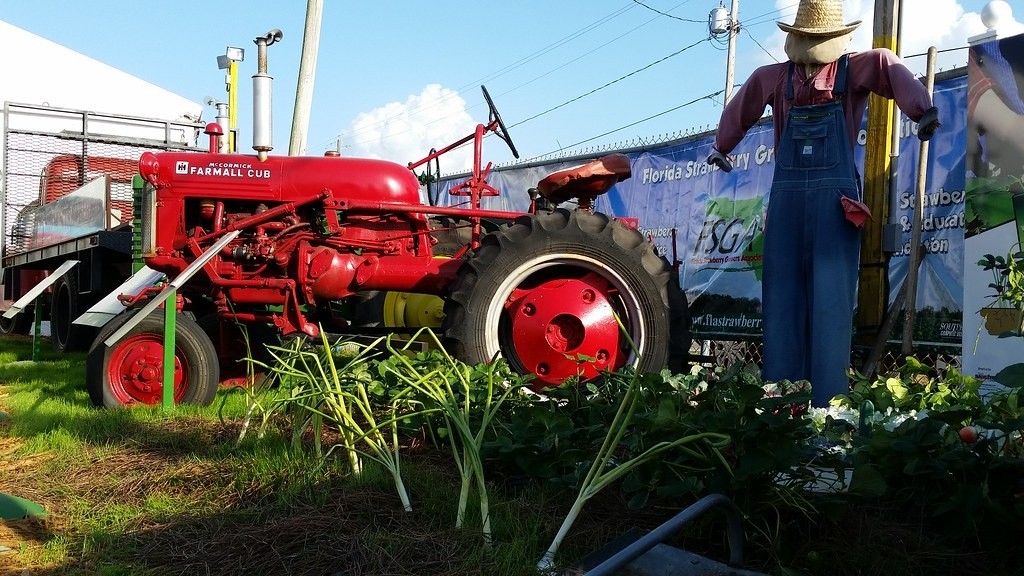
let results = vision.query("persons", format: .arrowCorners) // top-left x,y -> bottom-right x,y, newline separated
707,0 -> 939,410
966,32 -> 1024,196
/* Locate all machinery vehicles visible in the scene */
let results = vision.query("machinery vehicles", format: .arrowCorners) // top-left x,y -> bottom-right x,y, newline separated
88,80 -> 693,415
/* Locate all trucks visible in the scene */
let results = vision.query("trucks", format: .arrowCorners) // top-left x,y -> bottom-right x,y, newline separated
1,155 -> 139,354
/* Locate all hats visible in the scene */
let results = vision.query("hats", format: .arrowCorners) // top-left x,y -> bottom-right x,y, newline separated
774,0 -> 862,38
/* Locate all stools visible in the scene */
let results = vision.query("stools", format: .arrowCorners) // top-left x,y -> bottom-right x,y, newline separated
535,151 -> 632,215
